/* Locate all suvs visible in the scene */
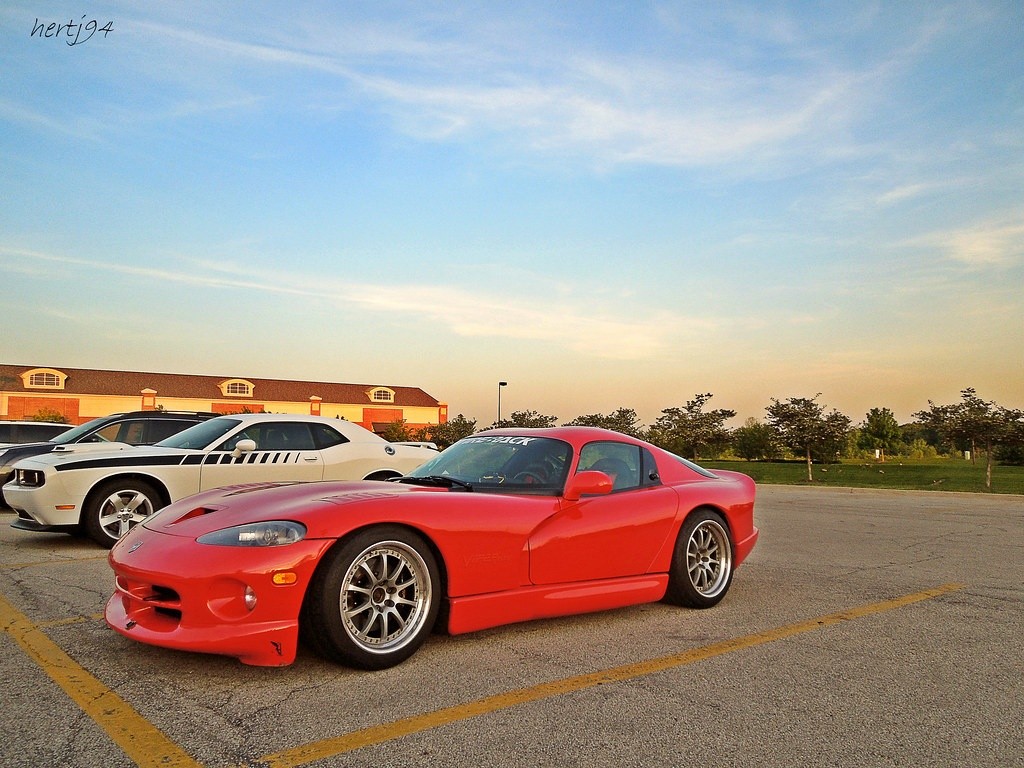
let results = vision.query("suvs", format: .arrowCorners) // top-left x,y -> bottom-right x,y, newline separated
0,411 -> 258,508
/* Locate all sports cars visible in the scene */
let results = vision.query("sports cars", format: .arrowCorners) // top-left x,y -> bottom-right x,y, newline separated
104,426 -> 759,671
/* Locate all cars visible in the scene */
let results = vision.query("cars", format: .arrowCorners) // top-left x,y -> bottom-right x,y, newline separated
3,413 -> 441,549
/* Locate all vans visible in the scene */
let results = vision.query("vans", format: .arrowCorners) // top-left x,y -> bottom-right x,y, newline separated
0,420 -> 111,457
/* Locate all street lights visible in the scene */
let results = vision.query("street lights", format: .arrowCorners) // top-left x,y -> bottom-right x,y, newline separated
498,382 -> 507,422
